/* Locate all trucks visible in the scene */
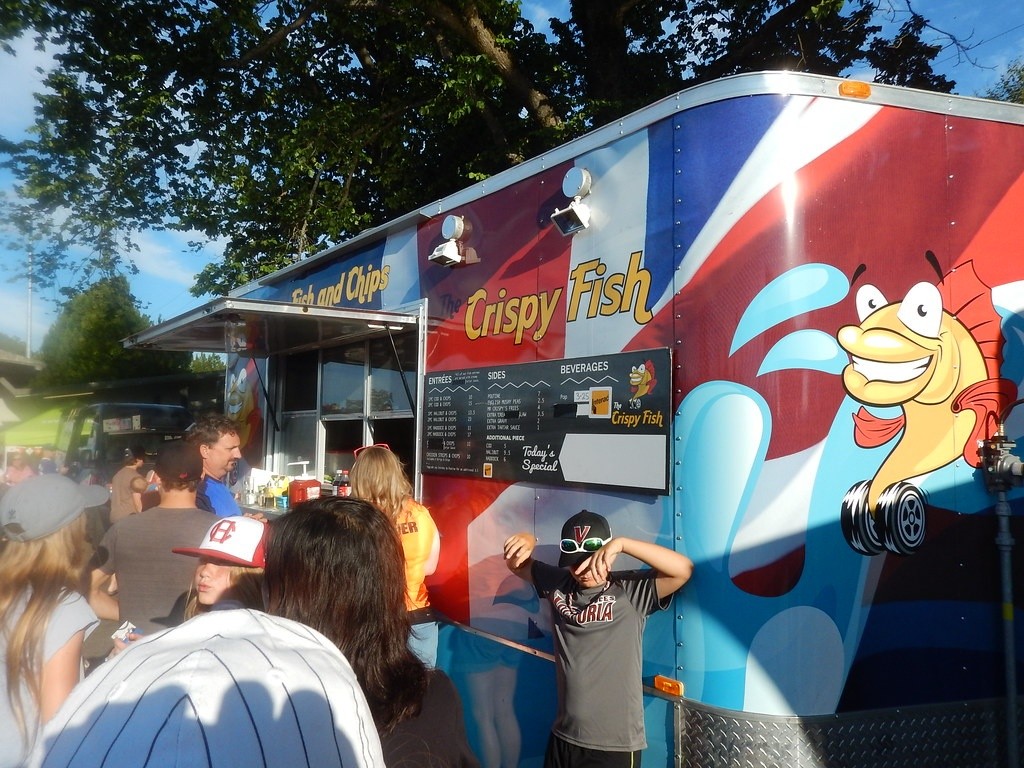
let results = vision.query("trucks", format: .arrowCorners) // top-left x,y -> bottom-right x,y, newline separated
125,70 -> 1024,768
52,400 -> 187,488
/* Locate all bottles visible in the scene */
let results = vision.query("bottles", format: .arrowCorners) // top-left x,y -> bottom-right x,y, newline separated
246,490 -> 255,505
261,482 -> 274,509
331,469 -> 342,495
288,473 -> 322,508
338,471 -> 352,497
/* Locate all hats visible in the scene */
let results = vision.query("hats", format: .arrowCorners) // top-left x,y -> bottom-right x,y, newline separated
0,473 -> 108,542
171,513 -> 269,569
558,509 -> 611,567
154,439 -> 203,481
122,445 -> 146,457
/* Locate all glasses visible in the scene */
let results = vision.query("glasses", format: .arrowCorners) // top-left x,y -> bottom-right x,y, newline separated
354,444 -> 389,460
559,528 -> 613,553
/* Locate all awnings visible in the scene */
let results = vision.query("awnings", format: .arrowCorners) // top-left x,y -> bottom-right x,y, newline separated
117,297 -> 419,431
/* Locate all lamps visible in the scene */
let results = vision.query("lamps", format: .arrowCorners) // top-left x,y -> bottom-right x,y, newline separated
427,215 -> 465,268
551,166 -> 592,236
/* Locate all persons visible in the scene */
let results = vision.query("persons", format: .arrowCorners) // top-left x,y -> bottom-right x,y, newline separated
257,493 -> 480,767
20,608 -> 384,767
504,510 -> 694,768
0,414 -> 480,768
1,474 -> 112,767
